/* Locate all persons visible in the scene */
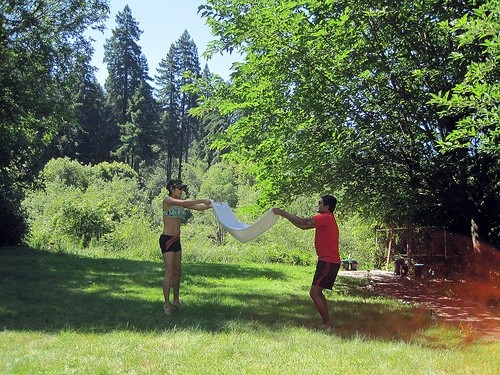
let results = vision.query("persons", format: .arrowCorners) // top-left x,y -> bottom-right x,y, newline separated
159,178 -> 222,317
272,195 -> 342,331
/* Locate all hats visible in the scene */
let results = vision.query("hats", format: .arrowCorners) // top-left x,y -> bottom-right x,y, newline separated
167,183 -> 187,188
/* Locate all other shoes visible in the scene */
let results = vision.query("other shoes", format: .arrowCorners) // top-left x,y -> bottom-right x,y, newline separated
164,302 -> 182,315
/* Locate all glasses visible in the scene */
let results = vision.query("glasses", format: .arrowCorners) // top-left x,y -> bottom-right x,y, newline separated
317,201 -> 323,204
175,186 -> 182,190
